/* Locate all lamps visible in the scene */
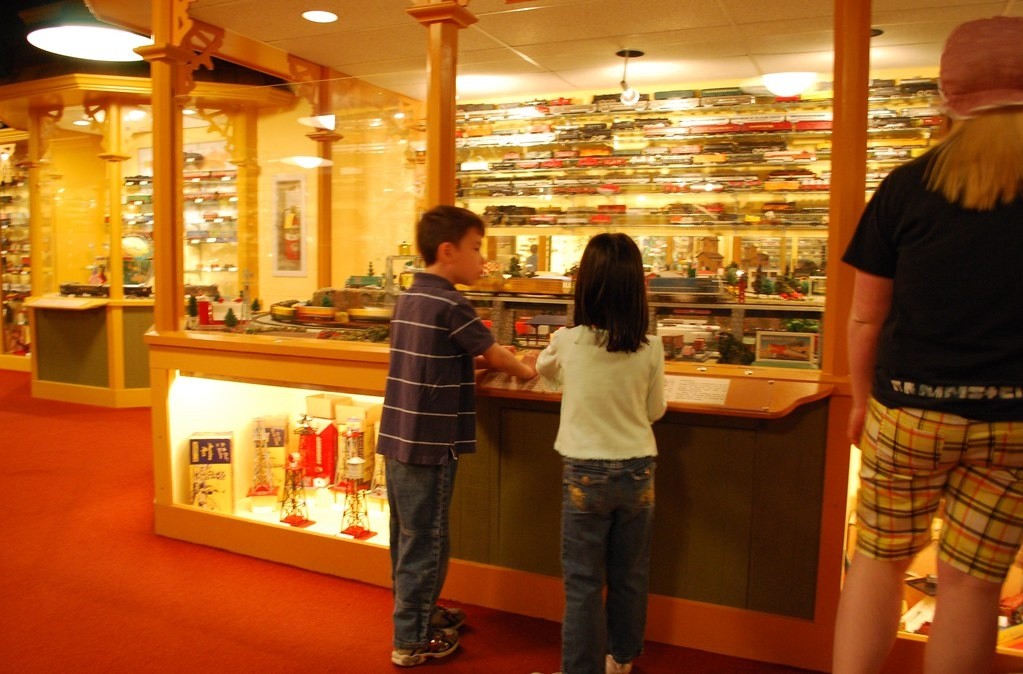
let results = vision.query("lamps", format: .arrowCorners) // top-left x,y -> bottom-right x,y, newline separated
619,80 -> 640,105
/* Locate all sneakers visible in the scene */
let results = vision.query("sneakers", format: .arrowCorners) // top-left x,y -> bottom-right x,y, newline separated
392,628 -> 460,666
429,606 -> 466,629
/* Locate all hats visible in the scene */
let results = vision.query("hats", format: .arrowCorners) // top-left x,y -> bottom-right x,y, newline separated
939,15 -> 1023,115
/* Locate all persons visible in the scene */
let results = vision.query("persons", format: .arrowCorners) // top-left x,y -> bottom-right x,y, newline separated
829,16 -> 1022,674
376,204 -> 537,665
535,233 -> 667,674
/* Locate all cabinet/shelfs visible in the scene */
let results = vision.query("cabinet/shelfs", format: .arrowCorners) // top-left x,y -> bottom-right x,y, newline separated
123,169 -> 242,275
413,93 -> 945,239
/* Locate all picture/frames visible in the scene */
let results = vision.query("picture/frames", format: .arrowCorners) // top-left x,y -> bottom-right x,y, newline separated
755,331 -> 814,365
